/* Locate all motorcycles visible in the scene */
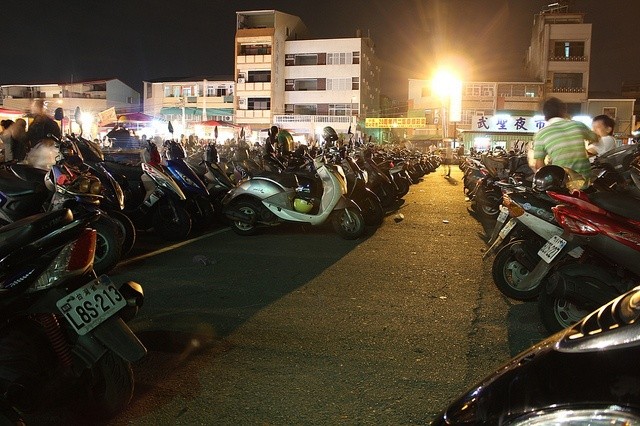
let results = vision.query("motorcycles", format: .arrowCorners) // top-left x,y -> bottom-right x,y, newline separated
0,208 -> 150,422
429,284 -> 640,426
458,137 -> 640,335
0,105 -> 443,267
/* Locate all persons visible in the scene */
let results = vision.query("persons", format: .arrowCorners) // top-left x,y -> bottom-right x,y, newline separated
587,114 -> 617,161
26,100 -> 60,147
106,120 -> 130,147
0,118 -> 26,162
533,97 -> 600,188
429,143 -> 436,153
263,126 -> 287,170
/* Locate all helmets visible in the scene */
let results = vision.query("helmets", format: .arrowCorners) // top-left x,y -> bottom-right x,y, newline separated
294,198 -> 313,213
532,165 -> 567,194
118,281 -> 143,309
321,126 -> 338,142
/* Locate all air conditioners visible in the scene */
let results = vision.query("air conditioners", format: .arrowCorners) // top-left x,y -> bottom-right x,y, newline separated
224,115 -> 232,122
238,78 -> 246,83
